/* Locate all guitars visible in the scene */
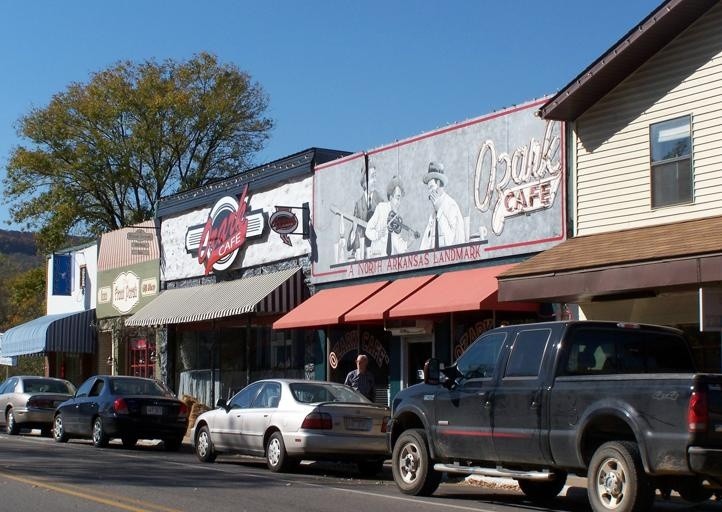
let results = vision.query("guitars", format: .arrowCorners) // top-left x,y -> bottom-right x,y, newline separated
329,205 -> 368,228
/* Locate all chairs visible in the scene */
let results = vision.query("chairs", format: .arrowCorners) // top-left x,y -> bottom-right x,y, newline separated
524,335 -> 625,373
267,396 -> 280,407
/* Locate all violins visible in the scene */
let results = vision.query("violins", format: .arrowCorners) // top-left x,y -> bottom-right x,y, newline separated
387,210 -> 420,239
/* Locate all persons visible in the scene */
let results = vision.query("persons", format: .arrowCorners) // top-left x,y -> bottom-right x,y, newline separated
346,159 -> 387,260
344,354 -> 376,405
363,176 -> 412,259
415,161 -> 468,251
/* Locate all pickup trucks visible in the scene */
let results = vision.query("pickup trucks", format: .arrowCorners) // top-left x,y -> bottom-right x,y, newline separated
384,320 -> 721,510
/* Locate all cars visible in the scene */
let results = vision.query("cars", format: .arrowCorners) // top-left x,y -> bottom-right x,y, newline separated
190,379 -> 392,470
53,375 -> 188,450
1,374 -> 77,436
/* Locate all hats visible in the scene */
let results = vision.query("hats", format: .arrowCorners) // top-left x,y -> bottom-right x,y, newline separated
422,162 -> 449,186
387,174 -> 406,200
359,164 -> 376,185
